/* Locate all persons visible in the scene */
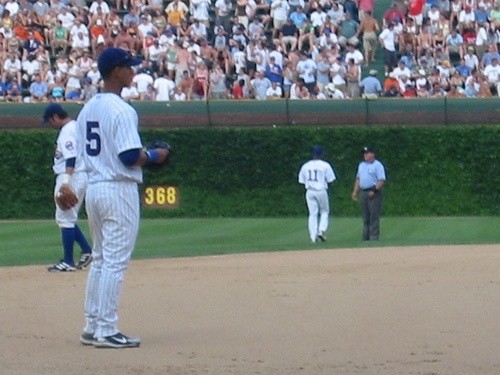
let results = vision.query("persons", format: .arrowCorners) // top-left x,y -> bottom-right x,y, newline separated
145,149 -> 159,166
0,0 -> 216,102
378,0 -> 500,99
298,144 -> 336,243
358,0 -> 376,22
210,0 -> 364,100
351,143 -> 386,241
40,103 -> 93,273
77,47 -> 170,349
355,10 -> 380,67
358,69 -> 383,100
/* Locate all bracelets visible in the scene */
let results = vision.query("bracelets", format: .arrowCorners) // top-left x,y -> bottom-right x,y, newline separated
63,174 -> 71,184
373,188 -> 378,193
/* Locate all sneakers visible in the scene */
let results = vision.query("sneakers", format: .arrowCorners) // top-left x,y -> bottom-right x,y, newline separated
93,333 -> 141,349
79,332 -> 95,345
47,260 -> 75,272
77,250 -> 93,268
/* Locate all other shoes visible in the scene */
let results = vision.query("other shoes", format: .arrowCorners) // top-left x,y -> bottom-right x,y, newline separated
319,234 -> 325,242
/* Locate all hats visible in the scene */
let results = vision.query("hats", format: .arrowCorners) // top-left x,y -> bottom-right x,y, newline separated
43,103 -> 61,125
313,146 -> 323,155
361,147 -> 374,154
97,49 -> 143,73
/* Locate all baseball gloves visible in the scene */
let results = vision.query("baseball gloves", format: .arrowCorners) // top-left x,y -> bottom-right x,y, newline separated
54,183 -> 80,212
142,142 -> 173,172
